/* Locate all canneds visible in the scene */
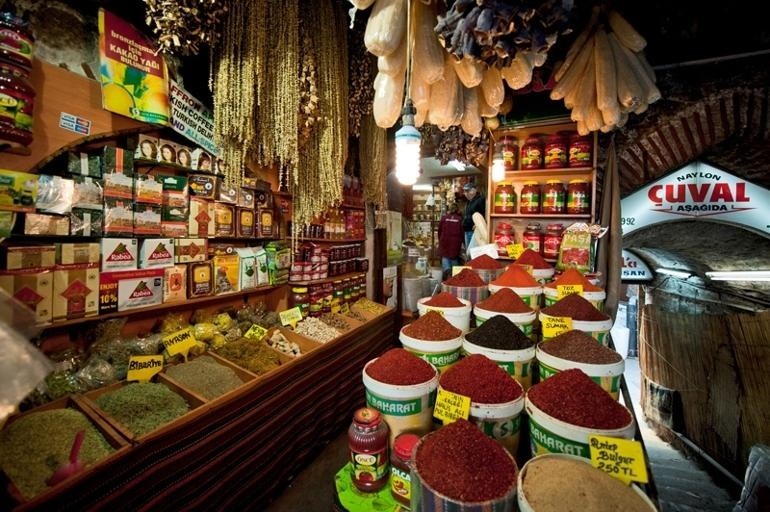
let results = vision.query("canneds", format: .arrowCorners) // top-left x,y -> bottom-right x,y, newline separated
287,244 -> 368,320
493,132 -> 592,259
389,435 -> 424,505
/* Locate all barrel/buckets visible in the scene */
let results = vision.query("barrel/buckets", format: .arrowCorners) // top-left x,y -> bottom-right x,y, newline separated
361,264 -> 659,512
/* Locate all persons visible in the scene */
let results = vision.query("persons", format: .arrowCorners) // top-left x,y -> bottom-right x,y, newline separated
197,152 -> 211,172
461,182 -> 486,251
436,202 -> 465,281
139,139 -> 158,162
177,148 -> 192,169
160,144 -> 176,164
216,159 -> 227,175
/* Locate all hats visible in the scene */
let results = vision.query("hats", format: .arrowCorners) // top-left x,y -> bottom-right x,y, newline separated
463,184 -> 472,190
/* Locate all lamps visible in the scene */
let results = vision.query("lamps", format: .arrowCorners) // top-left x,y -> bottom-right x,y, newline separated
393,0 -> 422,184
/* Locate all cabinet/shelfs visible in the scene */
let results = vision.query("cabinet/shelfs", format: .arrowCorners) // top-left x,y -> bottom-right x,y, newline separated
486,121 -> 598,264
0,55 -> 394,512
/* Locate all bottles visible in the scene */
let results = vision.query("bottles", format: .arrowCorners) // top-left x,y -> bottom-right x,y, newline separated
389,431 -> 421,508
303,208 -> 346,241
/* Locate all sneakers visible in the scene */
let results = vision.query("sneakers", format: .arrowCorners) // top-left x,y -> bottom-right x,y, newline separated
442,270 -> 450,281
